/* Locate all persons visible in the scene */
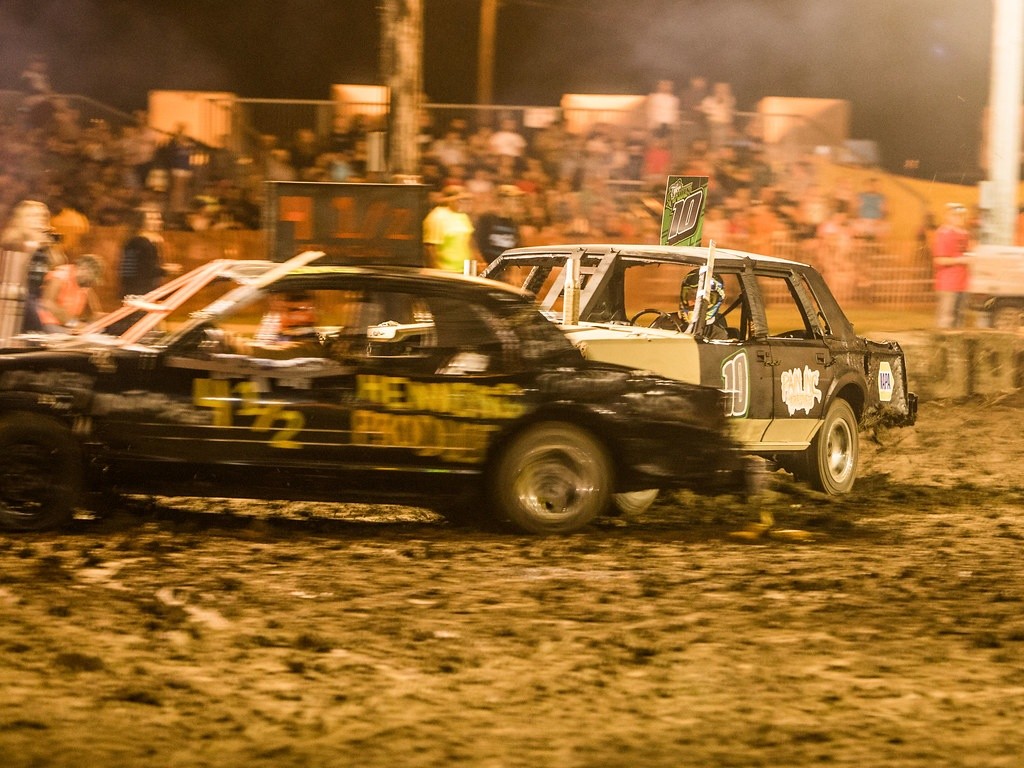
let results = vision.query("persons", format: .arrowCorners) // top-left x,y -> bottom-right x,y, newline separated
648,266 -> 735,340
33,251 -> 111,335
928,203 -> 976,332
114,200 -> 184,335
0,199 -> 55,341
197,292 -> 330,358
1,68 -> 993,271
19,207 -> 92,338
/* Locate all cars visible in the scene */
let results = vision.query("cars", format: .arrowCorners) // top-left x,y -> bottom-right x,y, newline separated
315,244 -> 918,516
0,257 -> 729,536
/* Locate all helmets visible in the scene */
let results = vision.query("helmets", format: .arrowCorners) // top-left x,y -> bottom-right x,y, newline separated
272,290 -> 318,327
77,257 -> 102,290
680,268 -> 725,323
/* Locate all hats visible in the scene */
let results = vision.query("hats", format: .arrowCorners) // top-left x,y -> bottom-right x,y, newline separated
497,184 -> 526,196
946,203 -> 968,214
436,185 -> 472,201
